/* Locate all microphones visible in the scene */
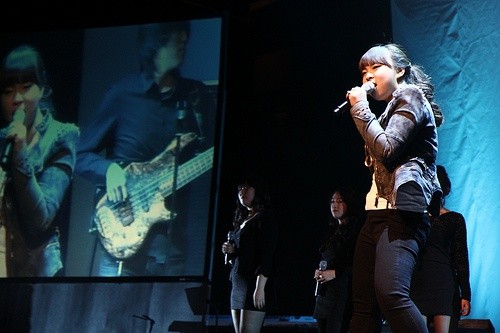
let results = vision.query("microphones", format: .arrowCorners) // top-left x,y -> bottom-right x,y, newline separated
2,108 -> 23,163
315,260 -> 327,297
224,231 -> 235,265
334,80 -> 375,114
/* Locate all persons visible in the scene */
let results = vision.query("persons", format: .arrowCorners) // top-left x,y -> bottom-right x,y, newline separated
76,20 -> 218,277
221,175 -> 277,333
309,189 -> 358,333
409,166 -> 471,333
345,43 -> 443,333
0,45 -> 82,277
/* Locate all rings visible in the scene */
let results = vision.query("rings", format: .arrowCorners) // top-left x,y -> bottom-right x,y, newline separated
347,91 -> 350,95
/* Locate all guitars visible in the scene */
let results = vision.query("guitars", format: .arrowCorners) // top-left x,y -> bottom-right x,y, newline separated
90,134 -> 214,263
